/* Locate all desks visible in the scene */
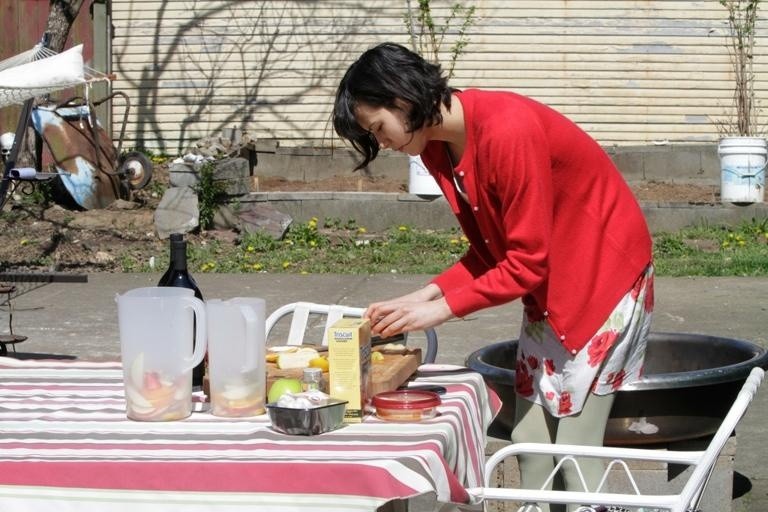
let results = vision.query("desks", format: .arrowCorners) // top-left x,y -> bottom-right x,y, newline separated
0,356 -> 503,512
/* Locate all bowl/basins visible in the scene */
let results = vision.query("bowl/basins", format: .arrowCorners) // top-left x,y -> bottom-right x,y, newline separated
462,331 -> 768,445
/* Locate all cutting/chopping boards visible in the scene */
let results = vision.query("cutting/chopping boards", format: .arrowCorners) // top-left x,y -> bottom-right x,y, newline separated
203,343 -> 422,398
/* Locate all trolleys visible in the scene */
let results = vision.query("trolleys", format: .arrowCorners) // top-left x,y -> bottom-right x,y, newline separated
27,76 -> 155,212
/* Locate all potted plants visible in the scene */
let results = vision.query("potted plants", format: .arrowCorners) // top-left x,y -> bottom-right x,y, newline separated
704,0 -> 768,203
402,0 -> 475,196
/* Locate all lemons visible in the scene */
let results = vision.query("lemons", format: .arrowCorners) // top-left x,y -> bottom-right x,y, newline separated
310,356 -> 329,371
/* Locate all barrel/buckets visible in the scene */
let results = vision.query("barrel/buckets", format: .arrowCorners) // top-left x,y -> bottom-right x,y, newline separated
716,136 -> 768,205
408,152 -> 443,195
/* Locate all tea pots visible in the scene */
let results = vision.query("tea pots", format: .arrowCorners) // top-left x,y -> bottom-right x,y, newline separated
114,286 -> 267,421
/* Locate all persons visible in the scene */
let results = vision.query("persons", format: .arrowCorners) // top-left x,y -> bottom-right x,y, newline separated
332,42 -> 655,512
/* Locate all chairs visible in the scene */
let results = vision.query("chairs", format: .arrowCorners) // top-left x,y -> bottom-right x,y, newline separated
266,301 -> 438,364
433,366 -> 764,512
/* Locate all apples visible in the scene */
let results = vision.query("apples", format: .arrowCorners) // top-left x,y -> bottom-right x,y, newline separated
269,378 -> 301,402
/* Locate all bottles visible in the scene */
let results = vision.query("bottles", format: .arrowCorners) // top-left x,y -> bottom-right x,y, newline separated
156,233 -> 206,392
